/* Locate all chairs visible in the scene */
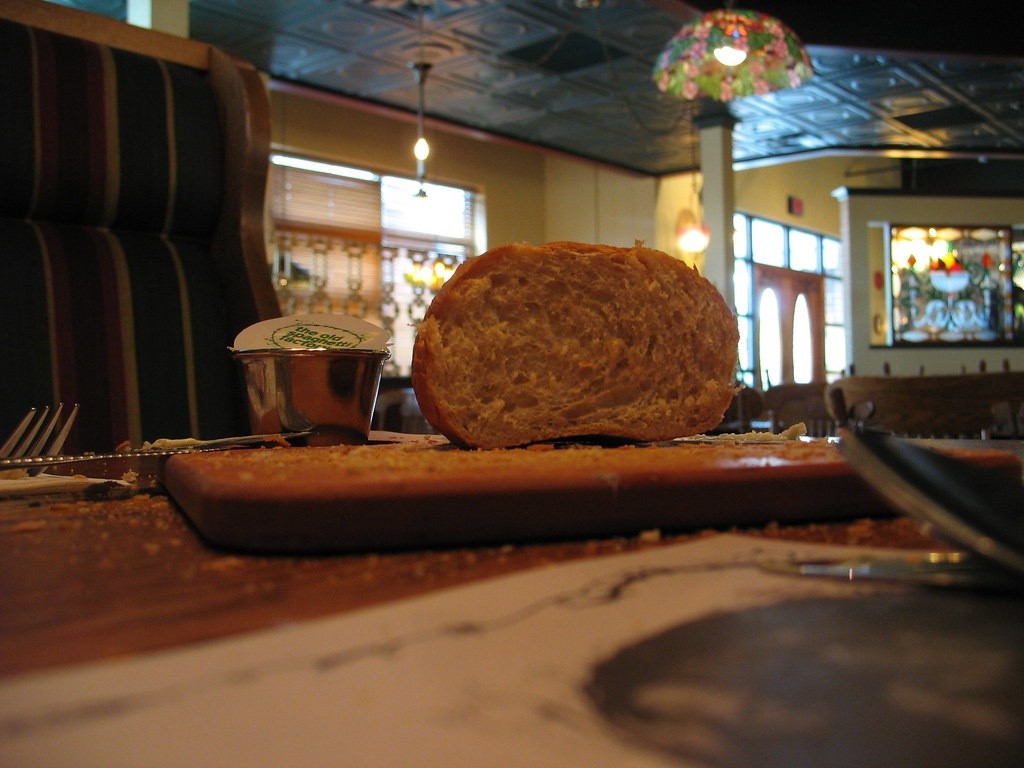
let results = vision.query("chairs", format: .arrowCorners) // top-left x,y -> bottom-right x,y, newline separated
720,369 -> 1023,441
0,0 -> 285,465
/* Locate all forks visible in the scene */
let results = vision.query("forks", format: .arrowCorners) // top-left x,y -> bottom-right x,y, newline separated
0,404 -> 78,478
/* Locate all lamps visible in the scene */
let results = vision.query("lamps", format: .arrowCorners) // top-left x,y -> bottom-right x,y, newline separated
648,7 -> 814,102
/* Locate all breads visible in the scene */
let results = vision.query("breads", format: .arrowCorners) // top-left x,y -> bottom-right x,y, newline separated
415,237 -> 740,448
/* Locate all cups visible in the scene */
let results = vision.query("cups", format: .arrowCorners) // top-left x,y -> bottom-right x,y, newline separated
235,349 -> 391,447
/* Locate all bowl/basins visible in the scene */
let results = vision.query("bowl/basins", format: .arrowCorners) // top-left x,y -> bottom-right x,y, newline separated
0,449 -> 242,493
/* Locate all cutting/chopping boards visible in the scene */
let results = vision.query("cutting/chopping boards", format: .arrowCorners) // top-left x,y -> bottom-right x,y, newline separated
166,431 -> 1023,556
